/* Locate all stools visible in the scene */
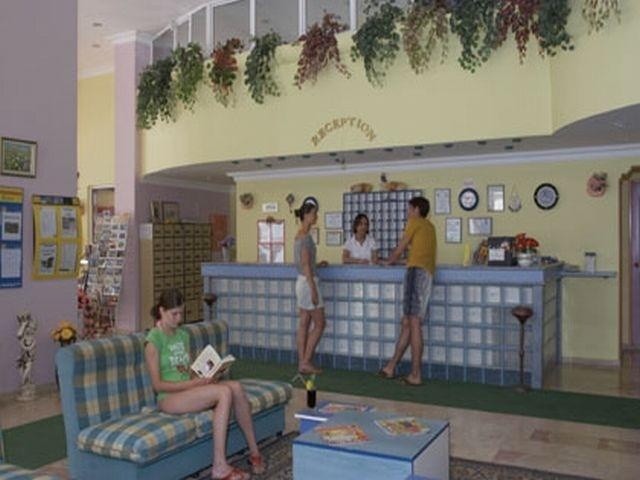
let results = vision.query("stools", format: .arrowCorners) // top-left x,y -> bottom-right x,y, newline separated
202,291 -> 218,319
510,307 -> 534,393
296,400 -> 377,432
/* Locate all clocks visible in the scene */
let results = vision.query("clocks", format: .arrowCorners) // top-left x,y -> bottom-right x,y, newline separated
304,197 -> 317,207
534,183 -> 559,209
456,188 -> 479,210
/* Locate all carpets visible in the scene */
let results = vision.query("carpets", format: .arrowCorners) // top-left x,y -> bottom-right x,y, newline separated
199,428 -> 600,480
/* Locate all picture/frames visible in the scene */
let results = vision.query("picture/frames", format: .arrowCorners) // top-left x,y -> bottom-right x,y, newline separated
446,218 -> 462,243
433,189 -> 451,216
310,212 -> 343,246
485,184 -> 506,214
0,135 -> 39,178
467,218 -> 493,236
163,200 -> 180,220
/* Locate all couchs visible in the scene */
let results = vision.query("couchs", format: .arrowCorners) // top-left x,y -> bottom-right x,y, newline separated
53,318 -> 293,480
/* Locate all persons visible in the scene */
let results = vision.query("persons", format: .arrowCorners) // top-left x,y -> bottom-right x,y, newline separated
377,196 -> 437,386
342,213 -> 378,266
291,203 -> 328,374
143,288 -> 268,479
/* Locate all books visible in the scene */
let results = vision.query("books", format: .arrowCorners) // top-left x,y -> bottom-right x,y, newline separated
317,401 -> 369,415
314,424 -> 372,448
190,343 -> 235,382
374,417 -> 429,437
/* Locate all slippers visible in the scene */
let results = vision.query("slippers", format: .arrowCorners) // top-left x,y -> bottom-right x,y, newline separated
376,368 -> 422,385
210,467 -> 249,480
247,454 -> 263,475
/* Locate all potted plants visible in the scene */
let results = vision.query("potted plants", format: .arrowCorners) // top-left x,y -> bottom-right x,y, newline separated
511,234 -> 542,270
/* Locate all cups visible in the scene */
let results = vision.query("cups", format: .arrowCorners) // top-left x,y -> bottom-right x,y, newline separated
516,253 -> 534,269
306,389 -> 317,410
